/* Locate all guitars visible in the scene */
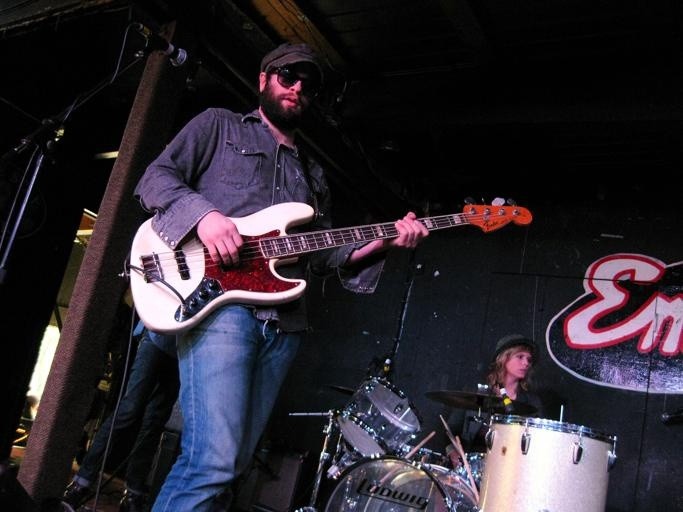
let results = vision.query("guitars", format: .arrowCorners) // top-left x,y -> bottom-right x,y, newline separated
129,198 -> 533,335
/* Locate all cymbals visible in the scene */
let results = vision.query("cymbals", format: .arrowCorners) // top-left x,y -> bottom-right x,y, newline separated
324,383 -> 356,396
423,390 -> 536,416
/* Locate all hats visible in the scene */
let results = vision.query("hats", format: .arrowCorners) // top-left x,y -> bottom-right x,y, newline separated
260,43 -> 334,85
491,334 -> 538,367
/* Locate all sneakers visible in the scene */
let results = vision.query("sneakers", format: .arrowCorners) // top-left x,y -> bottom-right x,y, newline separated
118,488 -> 146,512
255,447 -> 281,481
61,480 -> 90,512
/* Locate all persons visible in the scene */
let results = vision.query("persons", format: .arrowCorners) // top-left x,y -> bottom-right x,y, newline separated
60,320 -> 181,512
442,334 -> 544,453
134,44 -> 431,512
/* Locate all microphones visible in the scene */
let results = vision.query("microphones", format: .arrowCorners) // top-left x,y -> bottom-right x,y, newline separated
500,383 -> 516,413
384,358 -> 391,372
136,24 -> 187,67
660,410 -> 683,422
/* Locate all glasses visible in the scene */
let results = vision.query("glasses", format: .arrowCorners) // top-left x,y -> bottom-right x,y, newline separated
268,66 -> 319,98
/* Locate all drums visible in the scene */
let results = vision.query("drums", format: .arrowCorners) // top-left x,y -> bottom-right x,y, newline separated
476,412 -> 618,512
324,457 -> 478,512
337,376 -> 425,456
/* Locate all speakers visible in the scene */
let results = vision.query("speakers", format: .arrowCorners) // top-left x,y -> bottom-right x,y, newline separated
145,394 -> 319,512
